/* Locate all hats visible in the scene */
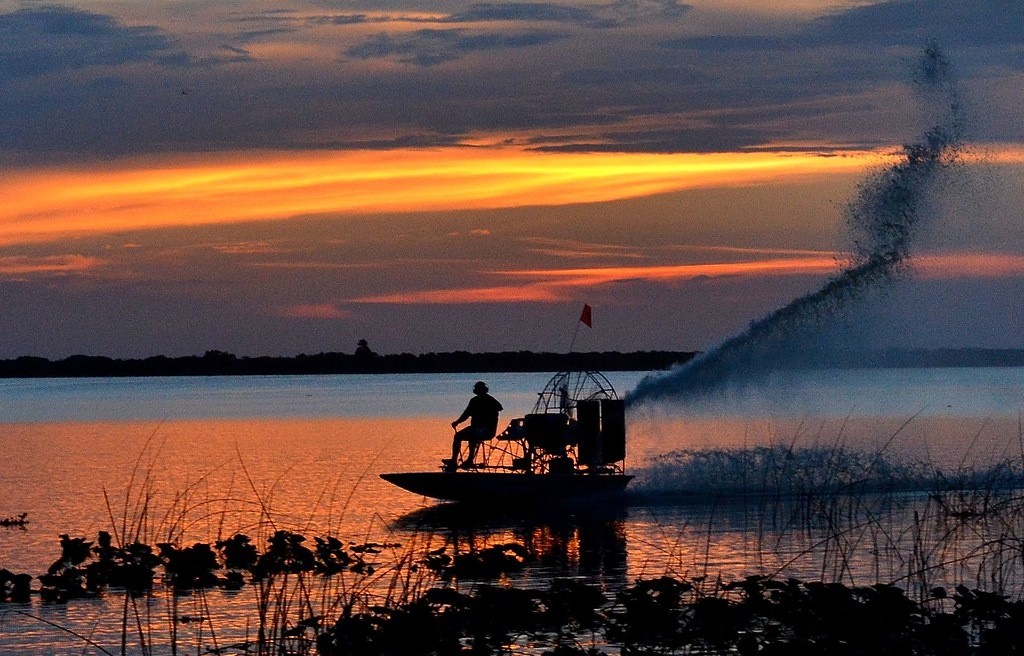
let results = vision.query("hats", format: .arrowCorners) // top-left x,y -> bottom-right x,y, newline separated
473,382 -> 489,394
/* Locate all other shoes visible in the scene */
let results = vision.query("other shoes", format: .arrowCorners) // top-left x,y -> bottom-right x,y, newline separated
459,459 -> 474,467
442,459 -> 458,468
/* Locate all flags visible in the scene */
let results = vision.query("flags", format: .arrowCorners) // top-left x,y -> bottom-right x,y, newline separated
580,304 -> 591,327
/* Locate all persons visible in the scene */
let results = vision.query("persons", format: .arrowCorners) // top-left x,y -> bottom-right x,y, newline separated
442,381 -> 503,469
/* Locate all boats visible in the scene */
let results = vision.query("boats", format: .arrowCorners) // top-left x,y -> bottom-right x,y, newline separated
377,357 -> 638,506
